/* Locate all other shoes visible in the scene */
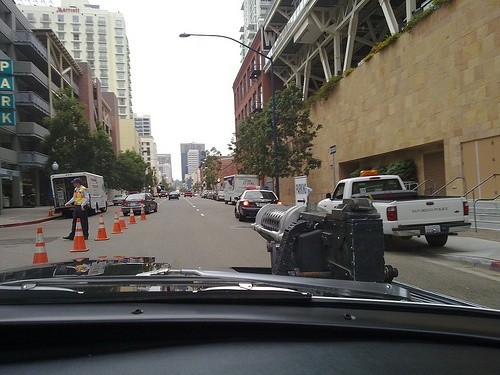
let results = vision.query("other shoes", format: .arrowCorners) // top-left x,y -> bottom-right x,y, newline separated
63,236 -> 71,239
84,234 -> 88,239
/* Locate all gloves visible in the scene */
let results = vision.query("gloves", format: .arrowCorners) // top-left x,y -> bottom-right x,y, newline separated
81,207 -> 85,211
65,203 -> 68,207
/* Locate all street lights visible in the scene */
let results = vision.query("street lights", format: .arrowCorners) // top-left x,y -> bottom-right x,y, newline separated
180,33 -> 278,200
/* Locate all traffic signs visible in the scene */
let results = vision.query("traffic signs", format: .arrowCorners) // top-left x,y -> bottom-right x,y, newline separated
294,176 -> 307,207
328,145 -> 337,154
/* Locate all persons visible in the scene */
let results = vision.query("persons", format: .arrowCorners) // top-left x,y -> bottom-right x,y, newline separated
62,178 -> 90,240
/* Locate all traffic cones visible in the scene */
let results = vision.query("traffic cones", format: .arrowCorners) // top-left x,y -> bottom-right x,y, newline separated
94,213 -> 110,240
118,210 -> 127,228
110,211 -> 123,234
140,206 -> 147,220
32,227 -> 49,264
70,218 -> 90,252
128,208 -> 137,224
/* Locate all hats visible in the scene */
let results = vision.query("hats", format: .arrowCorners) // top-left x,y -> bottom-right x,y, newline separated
70,178 -> 82,183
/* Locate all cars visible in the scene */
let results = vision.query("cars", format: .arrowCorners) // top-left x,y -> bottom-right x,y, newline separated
169,192 -> 179,200
193,190 -> 224,200
159,190 -> 168,198
234,186 -> 282,221
184,190 -> 192,197
113,194 -> 127,205
121,193 -> 158,216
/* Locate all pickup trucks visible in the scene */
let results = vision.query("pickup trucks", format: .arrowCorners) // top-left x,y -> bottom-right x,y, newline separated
315,169 -> 471,248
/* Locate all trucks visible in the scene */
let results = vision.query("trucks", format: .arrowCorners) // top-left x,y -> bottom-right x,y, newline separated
220,175 -> 260,204
50,172 -> 108,216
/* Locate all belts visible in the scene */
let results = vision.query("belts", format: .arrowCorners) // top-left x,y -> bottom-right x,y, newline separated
75,205 -> 81,206
